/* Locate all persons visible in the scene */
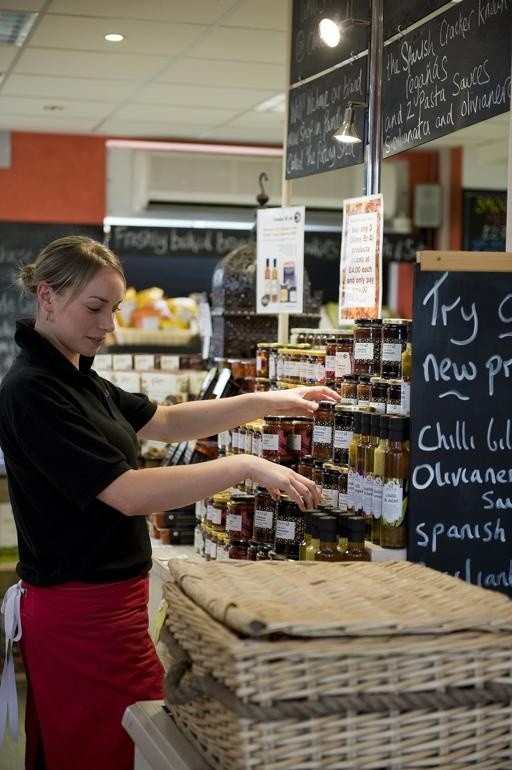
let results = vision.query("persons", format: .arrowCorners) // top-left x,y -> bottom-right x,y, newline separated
1,236 -> 342,770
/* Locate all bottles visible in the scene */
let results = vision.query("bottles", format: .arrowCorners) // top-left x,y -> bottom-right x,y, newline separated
256,343 -> 269,378
252,486 -> 289,545
352,412 -> 370,515
380,413 -> 409,546
262,259 -> 270,301
353,317 -> 382,375
292,417 -> 315,458
246,540 -> 263,561
280,416 -> 295,458
383,318 -> 408,380
325,339 -> 336,381
333,405 -> 351,466
195,494 -> 230,561
315,517 -> 342,561
299,509 -> 320,560
337,513 -> 356,553
338,468 -> 348,510
264,417 -> 286,459
269,343 -> 325,386
371,380 -> 388,409
314,403 -> 333,458
297,457 -> 314,478
346,412 -> 362,513
218,416 -> 262,461
374,416 -> 391,546
335,338 -> 353,383
272,258 -> 278,302
229,541 -> 248,560
279,285 -> 288,302
306,513 -> 328,561
387,382 -> 406,414
296,332 -> 353,344
275,493 -> 307,560
356,378 -> 370,404
322,466 -> 339,507
229,494 -> 255,540
290,287 -> 297,303
311,462 -> 324,485
362,413 -> 381,541
342,517 -> 371,561
256,545 -> 273,560
339,375 -> 358,406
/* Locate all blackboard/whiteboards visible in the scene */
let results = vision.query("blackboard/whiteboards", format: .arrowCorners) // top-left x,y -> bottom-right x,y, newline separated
461,187 -> 506,254
381,0 -> 512,158
279,57 -> 368,180
289,2 -> 371,84
100,216 -> 427,316
405,247 -> 512,599
382,1 -> 450,47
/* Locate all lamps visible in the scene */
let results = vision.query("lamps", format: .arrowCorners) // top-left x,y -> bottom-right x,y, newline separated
319,19 -> 371,48
332,102 -> 368,144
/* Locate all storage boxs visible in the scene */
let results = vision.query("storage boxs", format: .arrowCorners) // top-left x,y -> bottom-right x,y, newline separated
118,700 -> 211,769
